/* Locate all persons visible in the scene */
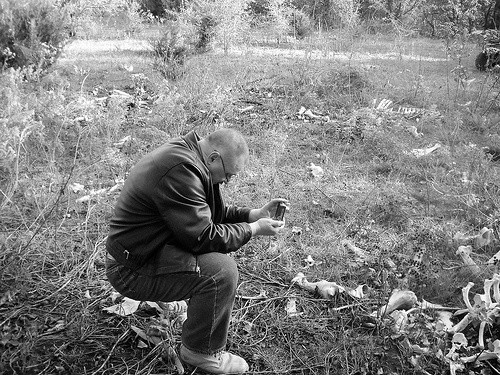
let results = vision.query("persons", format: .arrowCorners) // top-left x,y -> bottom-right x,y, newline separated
105,128 -> 291,375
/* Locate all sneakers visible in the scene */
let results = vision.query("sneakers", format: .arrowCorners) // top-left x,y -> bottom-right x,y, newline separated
179,343 -> 249,375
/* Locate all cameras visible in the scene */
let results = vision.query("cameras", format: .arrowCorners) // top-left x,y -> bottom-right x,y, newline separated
274,202 -> 291,222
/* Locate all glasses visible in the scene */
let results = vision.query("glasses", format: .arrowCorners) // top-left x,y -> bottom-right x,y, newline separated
215,149 -> 236,183
